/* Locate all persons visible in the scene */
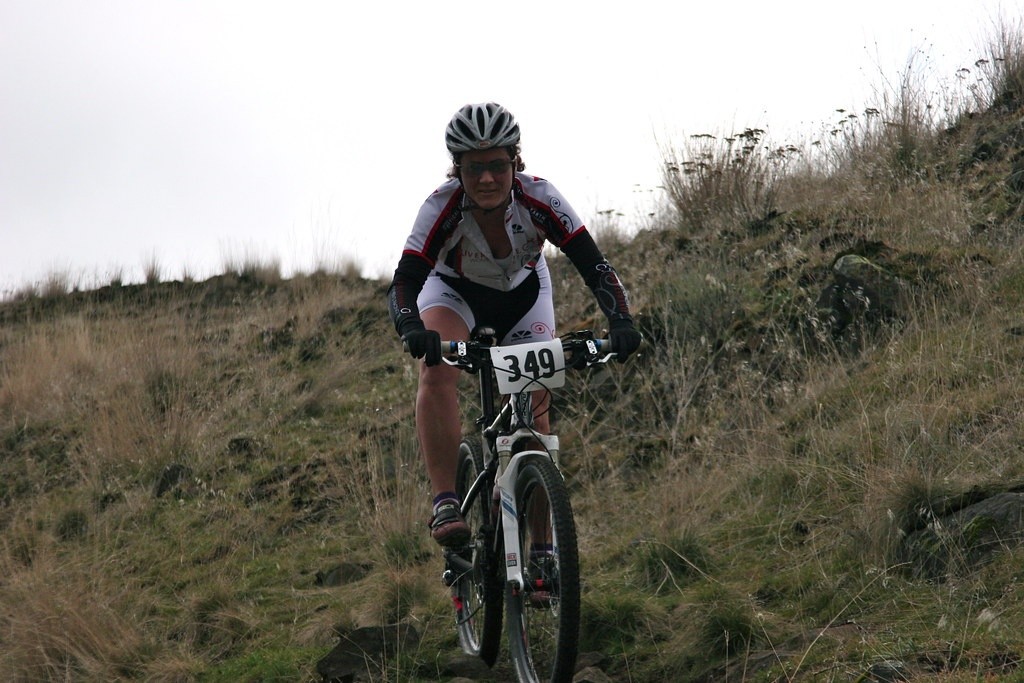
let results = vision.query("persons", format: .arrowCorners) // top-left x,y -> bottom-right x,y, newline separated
387,103 -> 644,585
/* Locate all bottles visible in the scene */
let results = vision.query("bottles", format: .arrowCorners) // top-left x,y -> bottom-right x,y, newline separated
490,464 -> 502,514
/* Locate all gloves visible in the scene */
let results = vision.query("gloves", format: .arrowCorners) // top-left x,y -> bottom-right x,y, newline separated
402,323 -> 442,368
603,313 -> 643,365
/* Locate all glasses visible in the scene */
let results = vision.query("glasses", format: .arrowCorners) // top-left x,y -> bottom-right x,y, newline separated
452,155 -> 520,175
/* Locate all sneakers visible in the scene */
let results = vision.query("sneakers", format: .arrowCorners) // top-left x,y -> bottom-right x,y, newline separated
425,503 -> 473,548
527,550 -> 561,611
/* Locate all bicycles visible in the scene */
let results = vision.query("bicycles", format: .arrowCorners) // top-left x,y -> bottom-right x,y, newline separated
401,328 -> 643,683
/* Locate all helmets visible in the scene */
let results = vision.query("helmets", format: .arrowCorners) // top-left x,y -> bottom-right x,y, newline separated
445,102 -> 522,153
